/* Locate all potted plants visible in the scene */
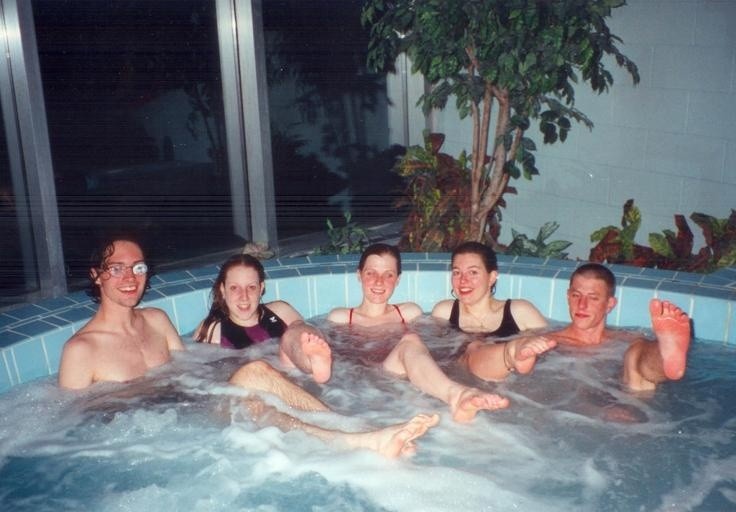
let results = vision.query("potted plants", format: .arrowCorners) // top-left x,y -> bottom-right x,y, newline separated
587,195 -> 652,266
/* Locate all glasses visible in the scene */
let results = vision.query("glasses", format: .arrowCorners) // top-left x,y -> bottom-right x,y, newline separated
98,263 -> 148,277
